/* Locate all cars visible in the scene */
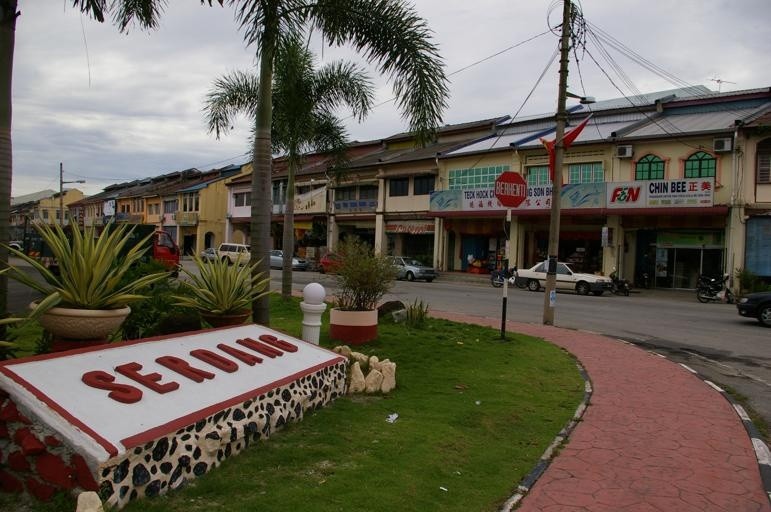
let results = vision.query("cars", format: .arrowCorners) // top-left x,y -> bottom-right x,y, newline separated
381,256 -> 437,283
516,260 -> 614,296
271,249 -> 309,271
737,289 -> 771,328
200,248 -> 218,264
318,250 -> 350,275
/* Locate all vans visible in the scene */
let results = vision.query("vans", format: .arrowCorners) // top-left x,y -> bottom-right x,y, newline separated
218,242 -> 252,267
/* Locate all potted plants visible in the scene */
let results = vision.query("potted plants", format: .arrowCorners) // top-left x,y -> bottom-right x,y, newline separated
0,202 -> 173,342
170,245 -> 280,326
320,233 -> 401,342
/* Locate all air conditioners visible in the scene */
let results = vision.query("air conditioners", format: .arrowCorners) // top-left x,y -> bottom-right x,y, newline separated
712,136 -> 732,152
616,145 -> 633,158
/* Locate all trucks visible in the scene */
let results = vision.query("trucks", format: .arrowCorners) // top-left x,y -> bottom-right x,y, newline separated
8,220 -> 181,284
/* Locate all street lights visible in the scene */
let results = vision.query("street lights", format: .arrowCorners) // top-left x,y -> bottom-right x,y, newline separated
543,88 -> 600,329
56,162 -> 87,228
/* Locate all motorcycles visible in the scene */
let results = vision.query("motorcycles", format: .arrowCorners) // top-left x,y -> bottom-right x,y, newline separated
696,274 -> 738,305
492,266 -> 529,289
609,268 -> 631,296
